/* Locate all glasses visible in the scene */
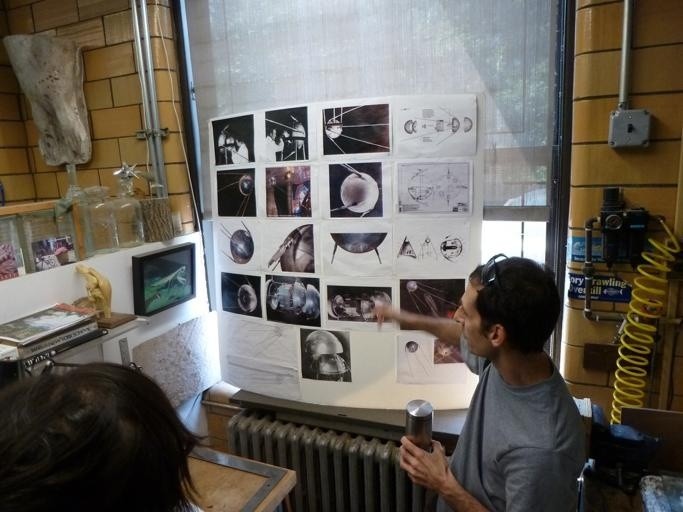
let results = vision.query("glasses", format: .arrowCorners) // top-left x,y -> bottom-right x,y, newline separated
484,253 -> 508,304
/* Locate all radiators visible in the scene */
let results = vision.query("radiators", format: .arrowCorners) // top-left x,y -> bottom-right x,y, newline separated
227,412 -> 446,512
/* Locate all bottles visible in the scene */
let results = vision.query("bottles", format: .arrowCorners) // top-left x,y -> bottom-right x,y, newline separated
405,398 -> 434,454
77,190 -> 145,257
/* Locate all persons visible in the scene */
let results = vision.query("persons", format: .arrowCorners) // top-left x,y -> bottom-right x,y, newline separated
366,252 -> 590,512
0,358 -> 212,512
227,135 -> 249,164
265,128 -> 284,162
